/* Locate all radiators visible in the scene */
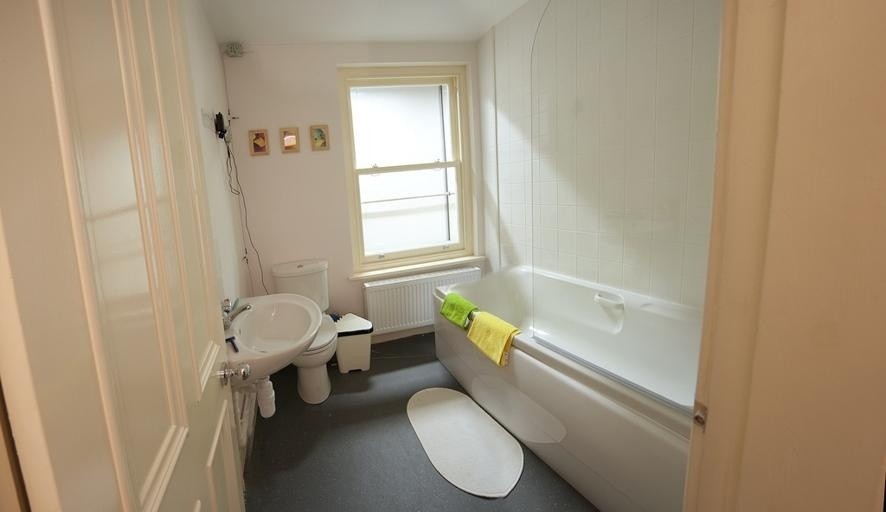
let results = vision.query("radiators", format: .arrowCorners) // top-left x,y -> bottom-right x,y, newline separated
361,267 -> 482,336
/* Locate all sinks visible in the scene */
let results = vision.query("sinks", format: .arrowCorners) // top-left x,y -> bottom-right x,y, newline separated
225,293 -> 323,384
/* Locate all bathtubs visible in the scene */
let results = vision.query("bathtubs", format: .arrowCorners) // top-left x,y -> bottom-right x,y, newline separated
434,267 -> 702,512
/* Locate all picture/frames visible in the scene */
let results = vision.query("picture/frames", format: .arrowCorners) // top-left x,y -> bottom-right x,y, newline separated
248,125 -> 329,156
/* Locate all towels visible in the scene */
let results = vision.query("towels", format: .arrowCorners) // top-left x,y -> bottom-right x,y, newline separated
439,293 -> 521,367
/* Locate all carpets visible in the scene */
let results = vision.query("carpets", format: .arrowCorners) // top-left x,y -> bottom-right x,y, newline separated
406,387 -> 525,498
471,376 -> 566,443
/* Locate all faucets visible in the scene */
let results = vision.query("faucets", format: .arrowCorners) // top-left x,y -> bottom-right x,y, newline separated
229,303 -> 251,320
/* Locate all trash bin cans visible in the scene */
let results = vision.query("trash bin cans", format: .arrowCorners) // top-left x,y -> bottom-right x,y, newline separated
335,312 -> 374,374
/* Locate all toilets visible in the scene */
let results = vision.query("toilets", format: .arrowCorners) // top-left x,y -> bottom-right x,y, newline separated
271,259 -> 337,405
